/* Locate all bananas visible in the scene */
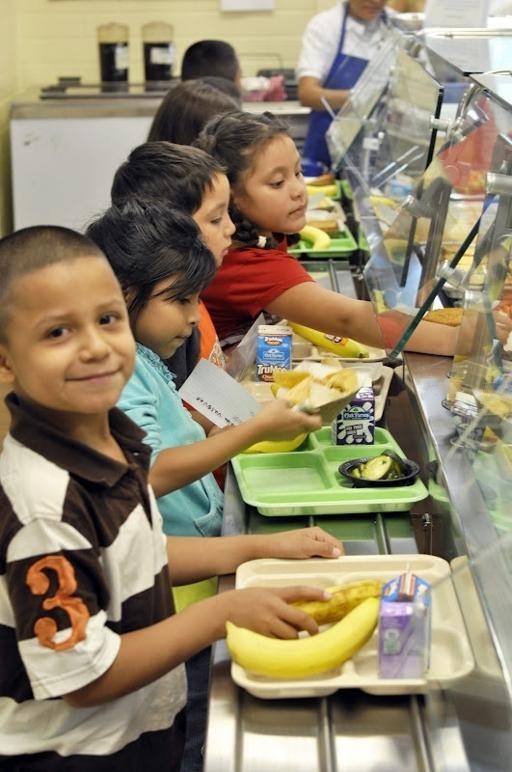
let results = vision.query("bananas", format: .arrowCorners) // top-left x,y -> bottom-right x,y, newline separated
284,580 -> 386,631
297,223 -> 330,250
243,431 -> 313,457
224,595 -> 378,681
288,321 -> 369,358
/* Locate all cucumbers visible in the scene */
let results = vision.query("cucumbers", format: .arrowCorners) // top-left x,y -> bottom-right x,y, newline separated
353,450 -> 408,479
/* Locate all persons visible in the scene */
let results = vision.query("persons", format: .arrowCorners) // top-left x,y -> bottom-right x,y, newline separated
190,109 -> 512,357
145,77 -> 237,145
295,0 -> 406,177
0,225 -> 346,772
181,39 -> 241,96
110,141 -> 237,411
82,197 -> 322,537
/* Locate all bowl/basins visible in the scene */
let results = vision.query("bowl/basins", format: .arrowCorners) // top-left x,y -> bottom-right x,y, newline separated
395,12 -> 426,30
337,455 -> 421,486
